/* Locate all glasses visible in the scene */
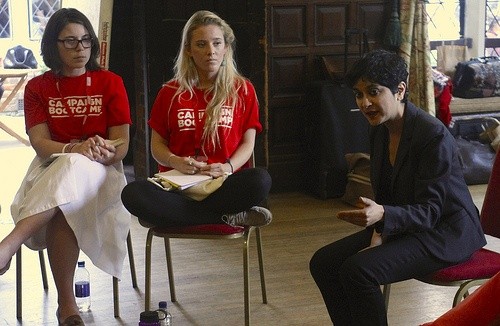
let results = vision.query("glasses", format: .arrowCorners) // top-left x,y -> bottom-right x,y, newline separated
56,35 -> 93,50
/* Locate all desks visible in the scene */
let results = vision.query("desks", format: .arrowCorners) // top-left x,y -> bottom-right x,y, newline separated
0,69 -> 40,147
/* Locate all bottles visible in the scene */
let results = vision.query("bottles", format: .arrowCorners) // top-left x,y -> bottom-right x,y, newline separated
74,261 -> 91,312
139,308 -> 167,326
157,301 -> 172,326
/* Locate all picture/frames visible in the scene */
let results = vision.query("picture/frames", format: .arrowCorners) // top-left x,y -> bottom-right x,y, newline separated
28,0 -> 62,41
0,0 -> 12,38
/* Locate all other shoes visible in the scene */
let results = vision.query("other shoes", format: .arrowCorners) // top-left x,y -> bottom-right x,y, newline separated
56,308 -> 85,326
0,257 -> 12,276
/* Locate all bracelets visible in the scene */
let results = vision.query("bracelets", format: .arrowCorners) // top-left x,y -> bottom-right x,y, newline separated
62,143 -> 71,153
64,143 -> 77,153
226,159 -> 234,173
165,154 -> 175,167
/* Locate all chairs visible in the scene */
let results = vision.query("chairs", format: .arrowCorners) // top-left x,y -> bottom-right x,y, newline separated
16,220 -> 138,322
380,116 -> 500,326
144,147 -> 269,326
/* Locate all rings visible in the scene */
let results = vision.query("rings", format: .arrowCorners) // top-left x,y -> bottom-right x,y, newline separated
187,160 -> 192,165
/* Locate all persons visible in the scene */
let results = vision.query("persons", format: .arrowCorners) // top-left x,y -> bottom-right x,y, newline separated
309,51 -> 487,326
0,7 -> 133,326
121,10 -> 272,229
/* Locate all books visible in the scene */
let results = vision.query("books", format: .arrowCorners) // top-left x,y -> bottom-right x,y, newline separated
154,169 -> 212,190
39,139 -> 124,168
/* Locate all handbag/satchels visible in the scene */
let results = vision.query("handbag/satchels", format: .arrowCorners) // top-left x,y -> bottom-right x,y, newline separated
146,168 -> 228,201
4,47 -> 37,69
452,133 -> 495,184
451,56 -> 500,99
340,152 -> 375,209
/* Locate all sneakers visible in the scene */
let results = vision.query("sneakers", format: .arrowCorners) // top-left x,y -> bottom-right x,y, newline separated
221,205 -> 273,229
138,217 -> 159,229
